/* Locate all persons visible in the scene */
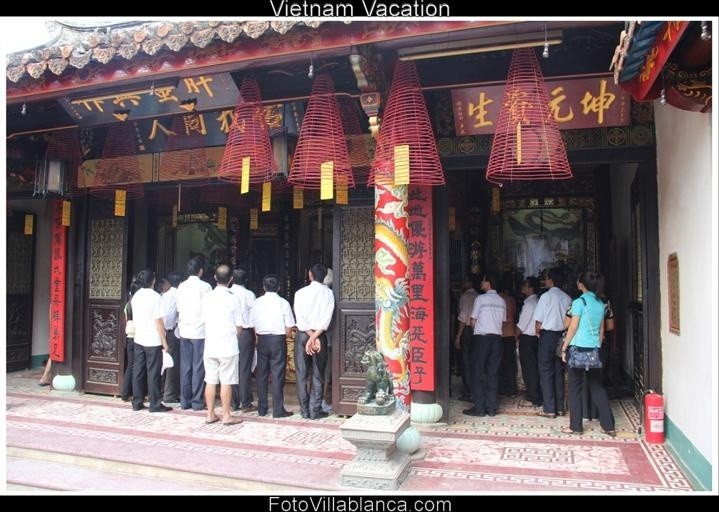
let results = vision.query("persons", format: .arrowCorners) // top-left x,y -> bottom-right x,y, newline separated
456,275 -> 480,401
565,291 -> 614,421
307,268 -> 332,411
38,358 -> 52,386
229,269 -> 259,411
294,264 -> 335,419
497,274 -> 517,398
154,278 -> 171,296
516,276 -> 541,407
253,274 -> 295,417
562,271 -> 616,436
176,259 -> 212,410
163,272 -> 181,402
463,272 -> 506,417
204,265 -> 243,425
122,282 -> 148,402
535,268 -> 573,417
131,270 -> 172,412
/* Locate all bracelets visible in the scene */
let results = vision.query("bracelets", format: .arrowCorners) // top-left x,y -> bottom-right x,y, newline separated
561,349 -> 567,353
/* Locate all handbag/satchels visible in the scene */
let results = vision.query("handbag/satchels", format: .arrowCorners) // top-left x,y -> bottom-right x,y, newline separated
568,298 -> 602,368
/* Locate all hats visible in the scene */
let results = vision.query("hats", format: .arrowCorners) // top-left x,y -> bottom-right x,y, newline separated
160,351 -> 174,377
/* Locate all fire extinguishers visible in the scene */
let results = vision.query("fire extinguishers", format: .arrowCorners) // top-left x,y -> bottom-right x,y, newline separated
638,388 -> 665,444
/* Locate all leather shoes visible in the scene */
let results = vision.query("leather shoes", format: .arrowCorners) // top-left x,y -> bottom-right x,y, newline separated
462,407 -> 485,416
231,406 -> 239,412
132,405 -> 146,410
181,405 -> 191,409
259,409 -> 266,416
486,408 -> 497,416
149,403 -> 171,412
520,400 -> 539,407
510,392 -> 517,398
457,394 -> 469,401
311,411 -> 328,419
193,406 -> 207,409
163,398 -> 179,403
242,406 -> 258,412
273,412 -> 292,418
496,392 -> 505,399
302,412 -> 310,418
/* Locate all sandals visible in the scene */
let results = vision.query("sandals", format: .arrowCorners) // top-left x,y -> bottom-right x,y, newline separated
536,410 -> 556,418
142,398 -> 149,402
37,378 -> 49,388
561,428 -> 582,435
604,429 -> 615,436
224,417 -> 242,425
207,416 -> 219,424
121,397 -> 131,402
556,409 -> 564,415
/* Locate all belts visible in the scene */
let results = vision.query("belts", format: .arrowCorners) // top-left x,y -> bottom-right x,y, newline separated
540,330 -> 565,335
